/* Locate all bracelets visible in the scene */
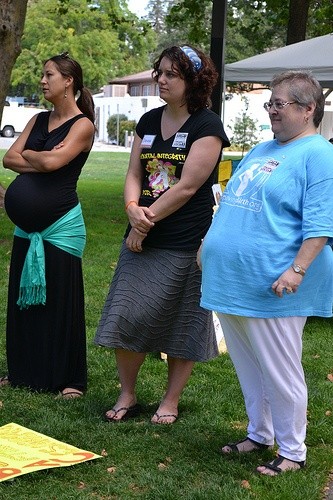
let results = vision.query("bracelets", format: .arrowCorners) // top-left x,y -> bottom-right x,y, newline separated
126,201 -> 138,210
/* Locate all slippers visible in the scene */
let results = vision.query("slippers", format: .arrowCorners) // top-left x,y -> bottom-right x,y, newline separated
0,376 -> 11,385
59,391 -> 82,400
104,404 -> 138,421
150,412 -> 178,425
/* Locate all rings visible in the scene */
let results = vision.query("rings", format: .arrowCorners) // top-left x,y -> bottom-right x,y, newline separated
286,287 -> 292,291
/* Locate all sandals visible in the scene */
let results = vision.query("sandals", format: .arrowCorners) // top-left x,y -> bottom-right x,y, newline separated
221,438 -> 274,456
254,454 -> 306,477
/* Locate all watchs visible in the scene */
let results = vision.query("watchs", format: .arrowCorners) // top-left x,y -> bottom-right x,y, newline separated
291,262 -> 305,276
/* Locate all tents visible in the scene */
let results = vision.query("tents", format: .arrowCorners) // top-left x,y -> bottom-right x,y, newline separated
225,32 -> 333,87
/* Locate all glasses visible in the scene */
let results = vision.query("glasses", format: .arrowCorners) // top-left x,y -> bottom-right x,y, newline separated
264,101 -> 299,111
61,52 -> 77,70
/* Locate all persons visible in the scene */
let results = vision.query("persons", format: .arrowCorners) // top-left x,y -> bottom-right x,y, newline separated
94,44 -> 231,424
197,70 -> 333,476
0,52 -> 98,400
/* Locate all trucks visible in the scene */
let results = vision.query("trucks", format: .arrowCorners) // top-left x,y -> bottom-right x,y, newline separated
0,106 -> 49,137
